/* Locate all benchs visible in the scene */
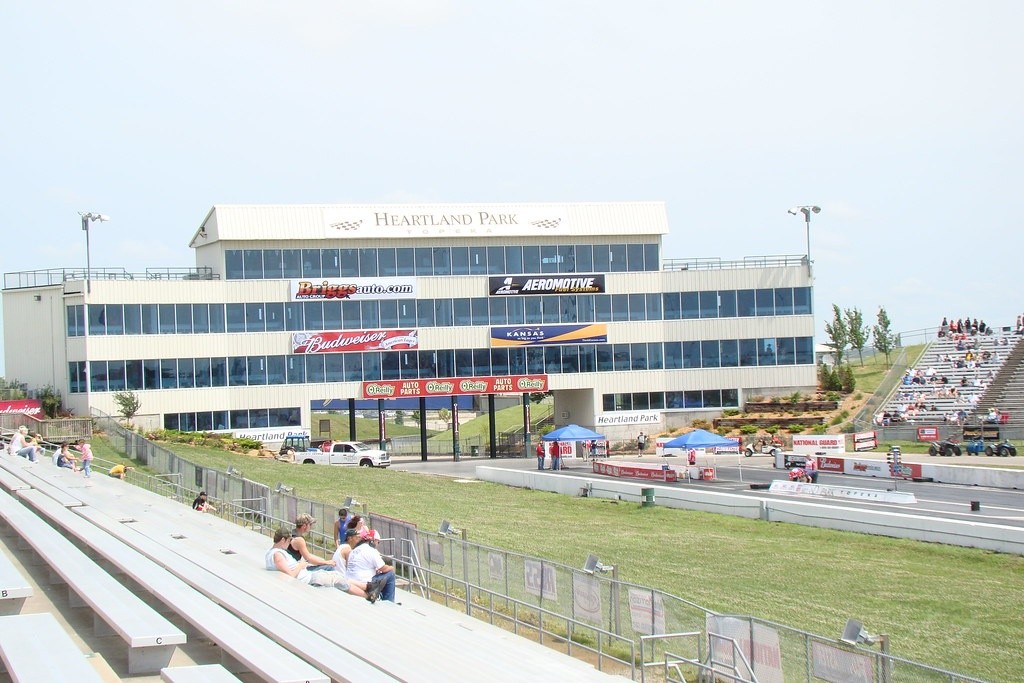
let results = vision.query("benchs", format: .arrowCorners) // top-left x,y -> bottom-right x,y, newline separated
0,449 -> 640,683
874,333 -> 1021,423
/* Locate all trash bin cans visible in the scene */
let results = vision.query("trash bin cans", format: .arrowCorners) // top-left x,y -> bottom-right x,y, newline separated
471,446 -> 479,456
641,487 -> 656,508
776,453 -> 784,469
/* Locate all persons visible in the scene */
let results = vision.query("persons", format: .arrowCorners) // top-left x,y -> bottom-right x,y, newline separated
789,454 -> 818,483
883,316 -> 1002,425
52,439 -> 93,477
688,448 -> 696,465
1003,337 -> 1009,346
288,447 -> 295,462
549,441 -> 559,470
637,432 -> 647,457
109,465 -> 127,479
193,491 -> 208,513
536,442 -> 545,470
265,508 -> 401,604
582,440 -> 598,461
1016,313 -> 1024,335
9,425 -> 45,462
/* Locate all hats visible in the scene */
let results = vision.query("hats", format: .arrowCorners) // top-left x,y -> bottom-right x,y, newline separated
345,529 -> 360,541
200,492 -> 208,496
369,530 -> 380,540
296,513 -> 316,525
275,527 -> 297,537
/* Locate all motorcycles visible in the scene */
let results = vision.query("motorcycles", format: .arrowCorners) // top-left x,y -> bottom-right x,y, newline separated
965,438 -> 1017,457
928,435 -> 962,456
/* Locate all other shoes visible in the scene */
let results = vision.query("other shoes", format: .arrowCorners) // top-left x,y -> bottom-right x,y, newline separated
367,576 -> 387,603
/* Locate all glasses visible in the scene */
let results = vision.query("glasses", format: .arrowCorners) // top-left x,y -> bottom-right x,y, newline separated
340,518 -> 345,519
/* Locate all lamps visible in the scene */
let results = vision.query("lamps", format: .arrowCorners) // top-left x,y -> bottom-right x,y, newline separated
34,296 -> 41,300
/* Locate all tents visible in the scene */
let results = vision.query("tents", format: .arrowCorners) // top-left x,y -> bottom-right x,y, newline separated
542,424 -> 606,466
663,428 -> 742,484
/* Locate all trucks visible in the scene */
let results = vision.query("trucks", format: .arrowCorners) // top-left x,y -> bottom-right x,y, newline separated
279,435 -> 392,456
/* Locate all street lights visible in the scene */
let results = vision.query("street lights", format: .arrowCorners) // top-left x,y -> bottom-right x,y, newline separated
79,210 -> 110,416
788,205 -> 821,278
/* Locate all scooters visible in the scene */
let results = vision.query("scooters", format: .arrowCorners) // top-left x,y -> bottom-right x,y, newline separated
744,435 -> 782,457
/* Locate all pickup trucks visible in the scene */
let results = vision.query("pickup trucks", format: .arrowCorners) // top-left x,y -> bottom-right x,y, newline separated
295,441 -> 391,468
